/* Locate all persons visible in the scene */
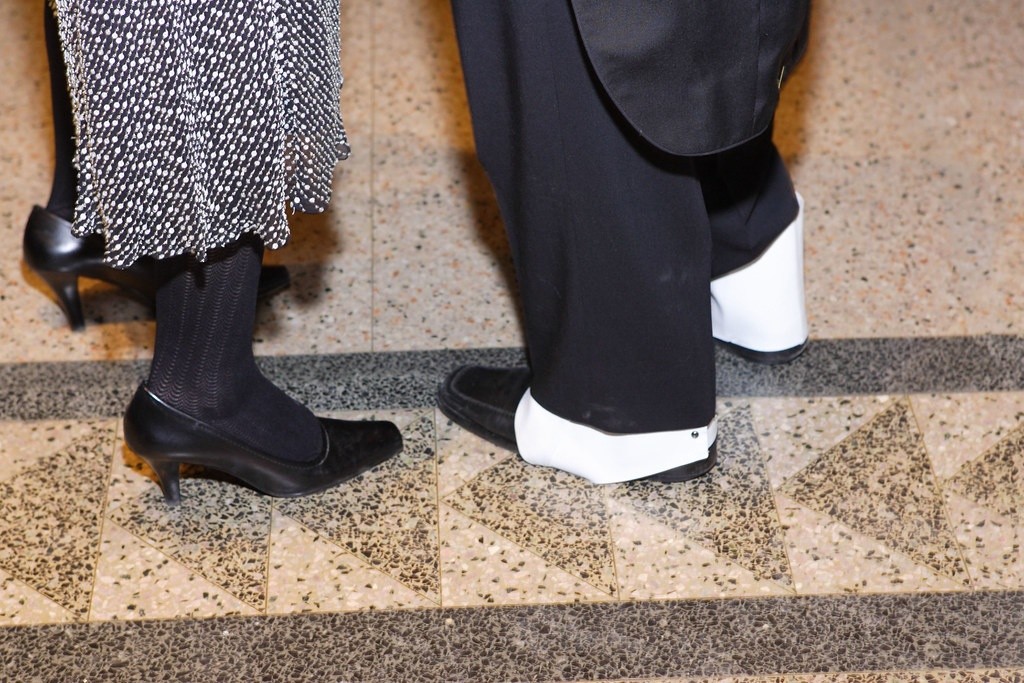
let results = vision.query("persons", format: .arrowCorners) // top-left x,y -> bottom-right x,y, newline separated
24,0 -> 403,505
438,0 -> 810,484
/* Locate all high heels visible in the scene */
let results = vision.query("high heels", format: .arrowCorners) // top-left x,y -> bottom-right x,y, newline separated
23,203 -> 290,330
123,380 -> 406,507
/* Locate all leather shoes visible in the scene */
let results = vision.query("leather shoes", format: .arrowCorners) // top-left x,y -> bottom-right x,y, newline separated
710,336 -> 809,364
436,365 -> 719,483
